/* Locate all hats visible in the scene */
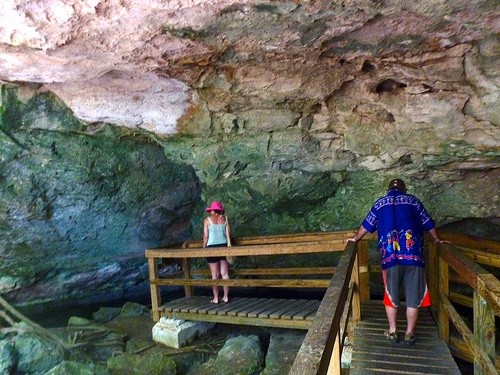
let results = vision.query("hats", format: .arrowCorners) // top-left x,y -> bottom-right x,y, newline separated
389,179 -> 405,189
206,202 -> 223,212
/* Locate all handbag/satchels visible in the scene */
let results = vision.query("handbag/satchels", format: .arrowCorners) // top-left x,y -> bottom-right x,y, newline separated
226,243 -> 236,264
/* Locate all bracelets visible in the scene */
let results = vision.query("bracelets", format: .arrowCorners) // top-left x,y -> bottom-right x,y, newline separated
434,238 -> 441,246
353,235 -> 359,242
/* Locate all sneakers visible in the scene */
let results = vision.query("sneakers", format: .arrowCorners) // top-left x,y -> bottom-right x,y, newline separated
404,334 -> 417,342
383,330 -> 398,342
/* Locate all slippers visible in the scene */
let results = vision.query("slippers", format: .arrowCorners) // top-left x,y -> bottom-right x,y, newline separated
210,299 -> 219,305
218,297 -> 228,304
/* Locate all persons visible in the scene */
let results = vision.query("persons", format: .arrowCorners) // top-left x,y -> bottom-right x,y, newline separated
201,201 -> 233,304
344,178 -> 454,348
